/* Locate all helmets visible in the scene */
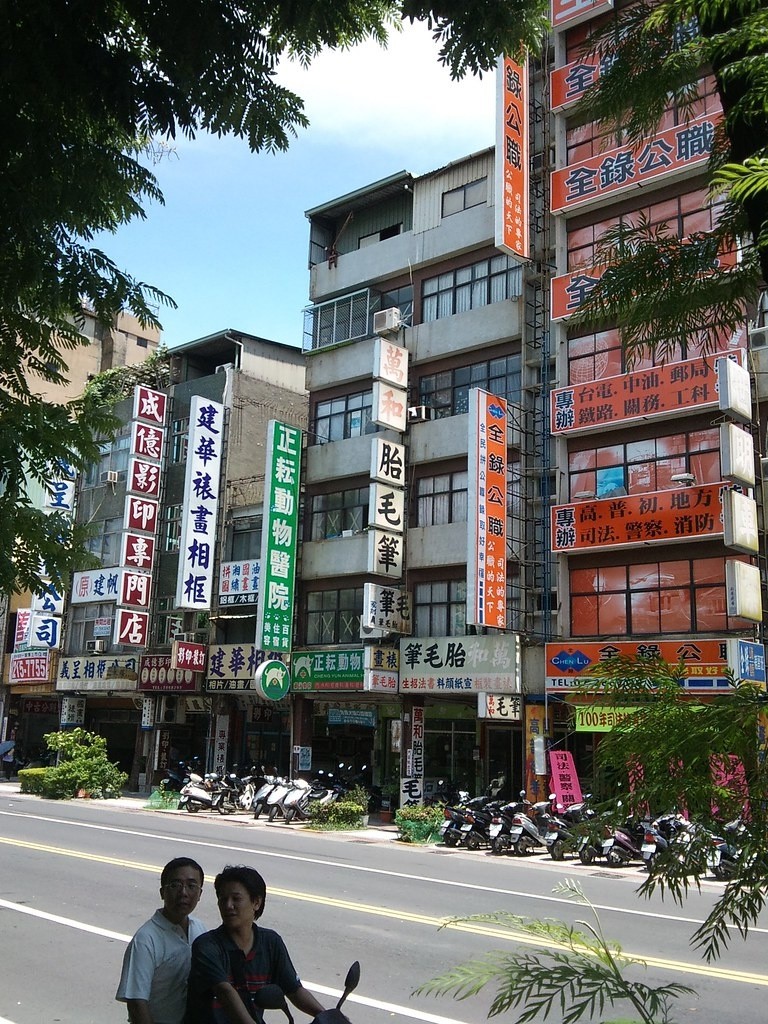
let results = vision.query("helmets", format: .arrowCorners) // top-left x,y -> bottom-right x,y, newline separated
209,773 -> 218,782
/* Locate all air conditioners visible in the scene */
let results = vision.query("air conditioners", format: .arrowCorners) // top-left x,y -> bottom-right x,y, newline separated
174,633 -> 188,642
215,362 -> 234,374
86,639 -> 103,653
751,327 -> 768,352
360,615 -> 389,638
374,307 -> 402,335
408,406 -> 435,423
100,471 -> 117,483
156,694 -> 176,724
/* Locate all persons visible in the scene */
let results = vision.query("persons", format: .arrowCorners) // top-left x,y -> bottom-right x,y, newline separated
2,748 -> 15,781
115,856 -> 210,1024
185,863 -> 350,1024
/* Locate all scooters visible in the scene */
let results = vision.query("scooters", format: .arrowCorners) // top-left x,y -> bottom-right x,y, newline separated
437,775 -> 768,883
253,960 -> 362,1024
163,756 -> 390,826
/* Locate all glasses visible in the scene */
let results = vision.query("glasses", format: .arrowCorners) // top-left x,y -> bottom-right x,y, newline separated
163,882 -> 201,890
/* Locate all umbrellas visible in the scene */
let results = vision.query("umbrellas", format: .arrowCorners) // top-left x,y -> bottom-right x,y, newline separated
0,740 -> 15,756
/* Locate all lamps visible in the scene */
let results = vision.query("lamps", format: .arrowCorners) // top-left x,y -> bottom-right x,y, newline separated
670,473 -> 696,487
574,491 -> 598,500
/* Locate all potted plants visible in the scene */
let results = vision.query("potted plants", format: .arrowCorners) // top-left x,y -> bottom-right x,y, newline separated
340,784 -> 371,826
378,784 -> 399,823
